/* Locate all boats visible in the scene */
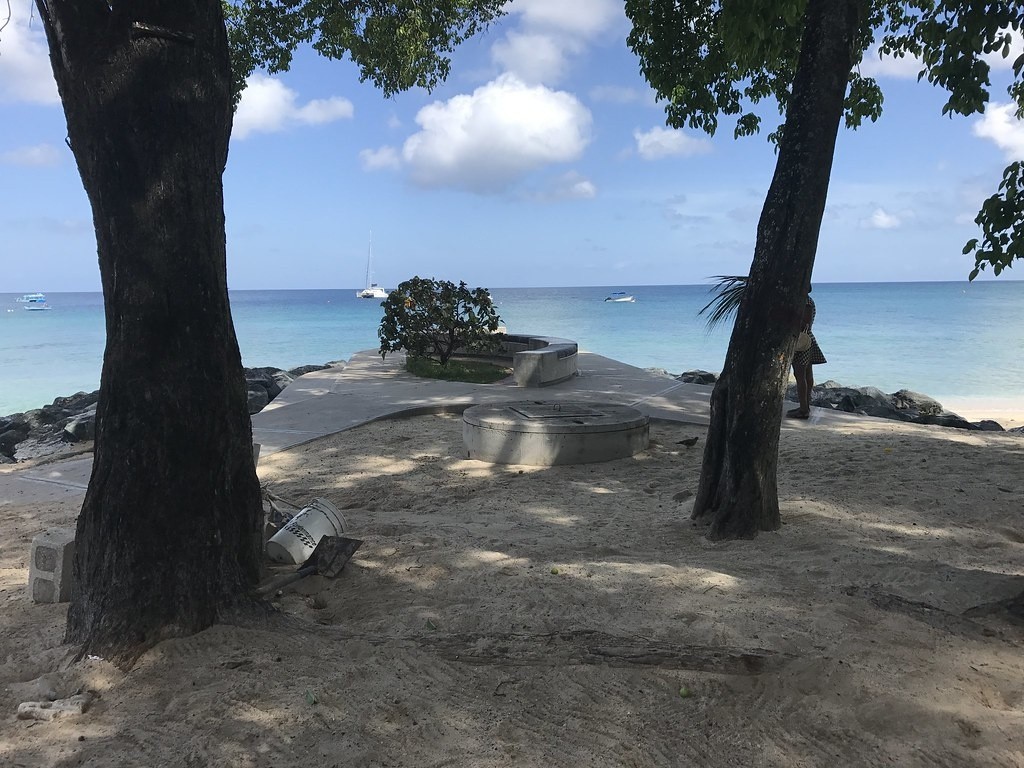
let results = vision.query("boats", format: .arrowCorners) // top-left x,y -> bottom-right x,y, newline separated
613,292 -> 625,294
605,296 -> 636,302
15,291 -> 47,303
23,305 -> 52,311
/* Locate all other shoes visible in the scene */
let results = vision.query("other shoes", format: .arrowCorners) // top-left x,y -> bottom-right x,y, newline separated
786,408 -> 811,420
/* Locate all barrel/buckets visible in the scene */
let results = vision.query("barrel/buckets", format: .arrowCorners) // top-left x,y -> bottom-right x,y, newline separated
267,497 -> 346,565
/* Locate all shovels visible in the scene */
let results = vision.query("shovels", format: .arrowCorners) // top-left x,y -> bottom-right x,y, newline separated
255,535 -> 364,596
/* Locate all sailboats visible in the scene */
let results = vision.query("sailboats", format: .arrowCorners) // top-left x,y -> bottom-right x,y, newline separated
356,226 -> 389,299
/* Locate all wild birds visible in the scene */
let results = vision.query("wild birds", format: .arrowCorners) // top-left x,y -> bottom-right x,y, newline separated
675,437 -> 699,449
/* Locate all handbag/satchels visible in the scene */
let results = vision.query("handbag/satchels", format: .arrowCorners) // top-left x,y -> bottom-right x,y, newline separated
794,325 -> 811,351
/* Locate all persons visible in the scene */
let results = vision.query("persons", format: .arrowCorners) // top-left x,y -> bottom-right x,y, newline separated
785,284 -> 828,420
